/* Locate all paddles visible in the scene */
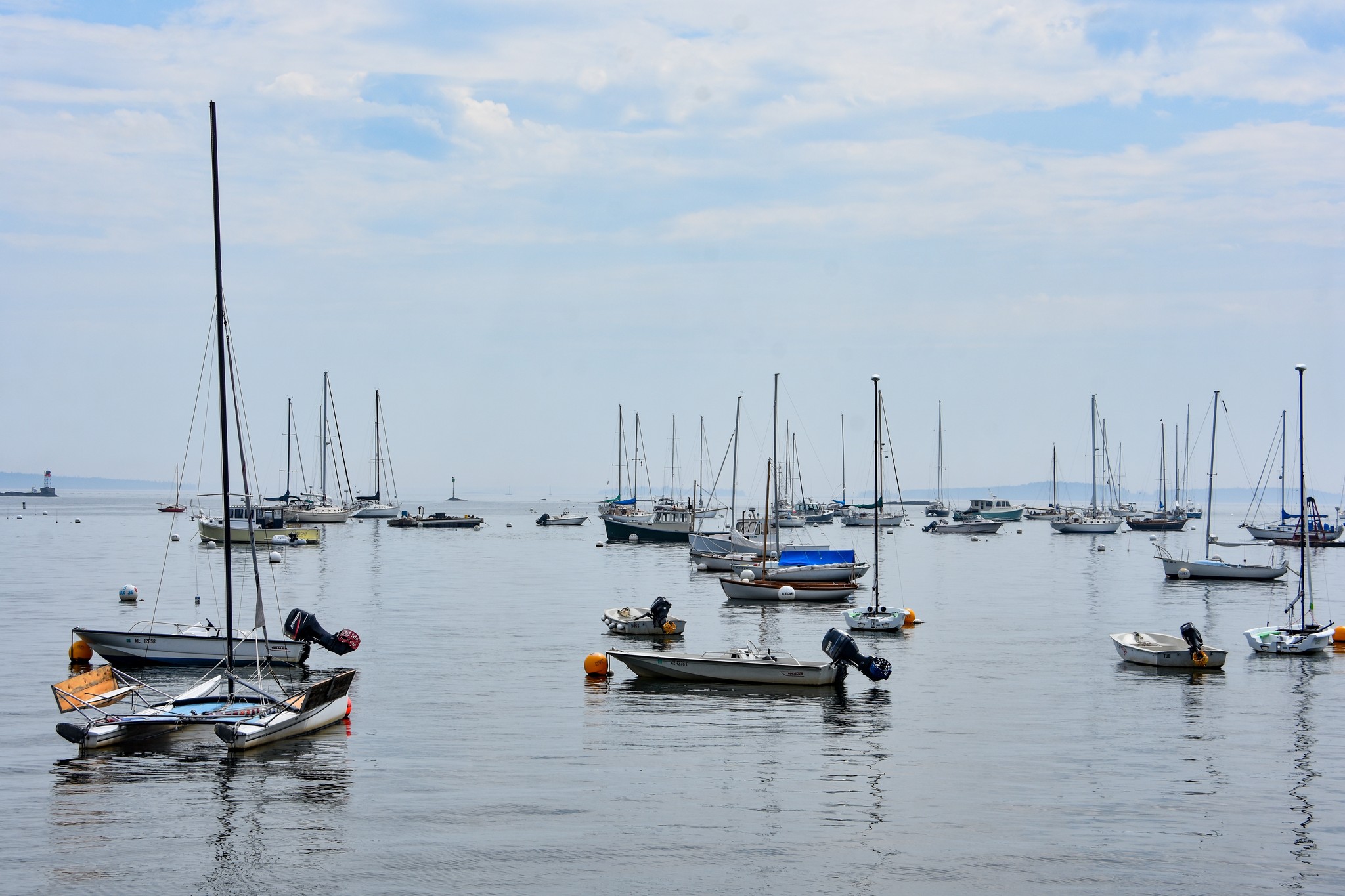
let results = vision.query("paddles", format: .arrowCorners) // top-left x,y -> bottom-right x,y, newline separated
1293,619 -> 1335,645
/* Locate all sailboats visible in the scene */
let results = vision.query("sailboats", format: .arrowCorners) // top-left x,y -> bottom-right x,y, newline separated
1022,395 -> 1204,533
598,373 -> 909,603
1238,408 -> 1345,548
1150,390 -> 1288,582
924,399 -> 952,517
1241,363 -> 1335,655
48,100 -> 355,757
156,372 -> 404,545
841,375 -> 913,632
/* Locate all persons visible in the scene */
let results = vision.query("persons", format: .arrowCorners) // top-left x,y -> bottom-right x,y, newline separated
939,518 -> 948,525
1030,510 -> 1036,515
1131,506 -> 1133,512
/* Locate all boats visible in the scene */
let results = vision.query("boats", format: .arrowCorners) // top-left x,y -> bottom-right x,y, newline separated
1108,621 -> 1229,669
603,627 -> 891,690
600,595 -> 688,638
536,507 -> 590,527
387,506 -> 484,529
923,497 -> 1028,535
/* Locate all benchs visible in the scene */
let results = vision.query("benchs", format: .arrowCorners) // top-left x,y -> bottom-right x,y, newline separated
1256,631 -> 1281,638
848,613 -> 861,618
276,670 -> 355,714
282,524 -> 302,529
892,613 -> 899,618
50,663 -> 145,725
